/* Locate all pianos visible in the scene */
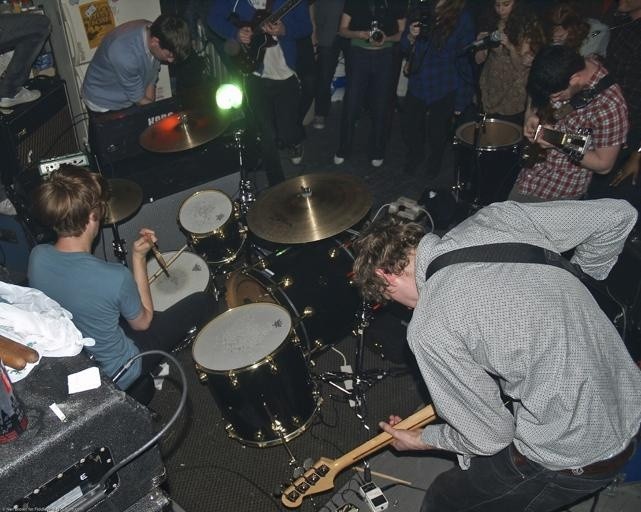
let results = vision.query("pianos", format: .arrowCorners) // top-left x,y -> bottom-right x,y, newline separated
96,97 -> 174,139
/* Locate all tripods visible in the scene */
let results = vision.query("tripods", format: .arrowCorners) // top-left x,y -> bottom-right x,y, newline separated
318,295 -> 388,420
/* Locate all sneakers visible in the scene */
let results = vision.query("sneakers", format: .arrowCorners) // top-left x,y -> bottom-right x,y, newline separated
313,116 -> 326,128
0,87 -> 41,108
289,142 -> 304,165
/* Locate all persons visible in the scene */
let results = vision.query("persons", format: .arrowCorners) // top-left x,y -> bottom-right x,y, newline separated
26,163 -> 221,391
207,1 -> 641,197
80,12 -> 200,155
348,198 -> 641,511
1,13 -> 53,107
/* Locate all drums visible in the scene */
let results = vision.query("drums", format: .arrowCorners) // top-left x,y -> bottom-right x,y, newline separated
227,241 -> 361,364
145,252 -> 217,346
191,302 -> 324,449
454,117 -> 523,206
178,191 -> 247,265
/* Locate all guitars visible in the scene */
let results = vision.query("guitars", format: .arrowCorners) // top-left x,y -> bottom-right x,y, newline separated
274,280 -> 634,508
519,118 -> 592,168
229,1 -> 304,74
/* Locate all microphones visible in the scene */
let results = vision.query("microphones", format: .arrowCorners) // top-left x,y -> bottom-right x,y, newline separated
465,30 -> 502,50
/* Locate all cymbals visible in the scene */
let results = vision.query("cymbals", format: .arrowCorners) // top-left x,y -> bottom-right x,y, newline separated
249,173 -> 375,244
101,179 -> 143,226
137,107 -> 229,153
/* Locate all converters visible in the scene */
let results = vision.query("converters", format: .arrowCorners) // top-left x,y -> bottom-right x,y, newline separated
340,365 -> 362,407
360,481 -> 388,512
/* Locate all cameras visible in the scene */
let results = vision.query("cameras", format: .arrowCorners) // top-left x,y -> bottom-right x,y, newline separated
369,19 -> 383,42
418,16 -> 430,32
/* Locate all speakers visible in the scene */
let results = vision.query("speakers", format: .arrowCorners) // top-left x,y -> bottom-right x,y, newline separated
0,75 -> 79,243
100,138 -> 239,203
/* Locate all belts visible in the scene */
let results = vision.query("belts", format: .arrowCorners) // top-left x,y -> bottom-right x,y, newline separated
510,441 -> 635,474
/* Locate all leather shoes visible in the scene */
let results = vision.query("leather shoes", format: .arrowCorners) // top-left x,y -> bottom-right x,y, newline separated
372,159 -> 384,167
333,154 -> 344,164
